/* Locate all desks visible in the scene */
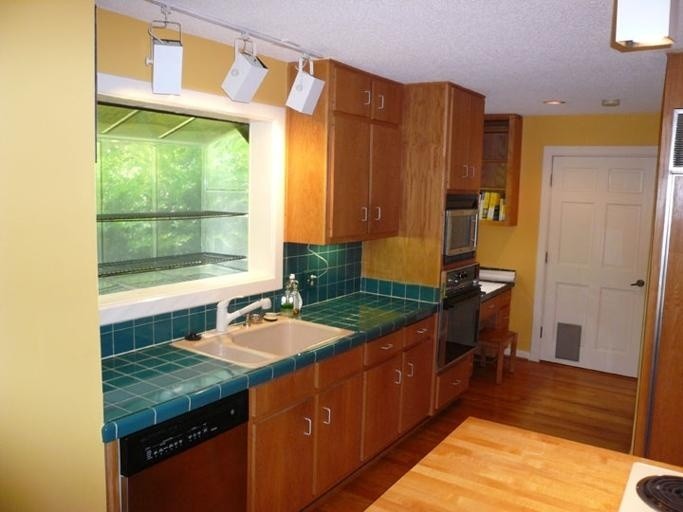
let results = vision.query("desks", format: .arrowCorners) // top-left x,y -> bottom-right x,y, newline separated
362,416 -> 683,512
479,268 -> 515,365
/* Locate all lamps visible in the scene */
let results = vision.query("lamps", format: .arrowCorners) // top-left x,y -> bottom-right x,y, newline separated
147,1 -> 325,116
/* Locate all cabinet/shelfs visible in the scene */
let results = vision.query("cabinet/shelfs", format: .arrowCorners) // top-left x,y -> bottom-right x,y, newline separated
484,305 -> 510,358
480,113 -> 522,226
286,60 -> 405,247
250,315 -> 434,511
360,84 -> 484,418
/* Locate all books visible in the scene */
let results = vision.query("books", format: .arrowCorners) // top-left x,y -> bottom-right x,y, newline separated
479,192 -> 504,221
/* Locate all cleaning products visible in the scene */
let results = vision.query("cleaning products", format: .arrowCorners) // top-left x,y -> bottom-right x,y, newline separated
280,274 -> 303,315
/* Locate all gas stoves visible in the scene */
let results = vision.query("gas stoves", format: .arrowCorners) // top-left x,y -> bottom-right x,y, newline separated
618,463 -> 683,512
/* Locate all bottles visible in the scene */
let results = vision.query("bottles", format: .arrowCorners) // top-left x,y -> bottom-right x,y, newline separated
281,273 -> 303,317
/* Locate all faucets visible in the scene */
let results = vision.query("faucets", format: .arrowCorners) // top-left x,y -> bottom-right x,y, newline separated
216,295 -> 272,331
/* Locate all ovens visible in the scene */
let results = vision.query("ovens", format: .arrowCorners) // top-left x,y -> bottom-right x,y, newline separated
433,262 -> 484,374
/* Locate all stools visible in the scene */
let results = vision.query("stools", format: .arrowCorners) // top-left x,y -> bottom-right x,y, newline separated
478,329 -> 517,385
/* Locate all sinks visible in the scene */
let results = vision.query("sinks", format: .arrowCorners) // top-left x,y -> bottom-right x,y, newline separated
169,312 -> 356,369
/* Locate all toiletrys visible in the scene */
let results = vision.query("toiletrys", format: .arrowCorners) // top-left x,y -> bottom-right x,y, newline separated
280,282 -> 293,314
291,279 -> 303,314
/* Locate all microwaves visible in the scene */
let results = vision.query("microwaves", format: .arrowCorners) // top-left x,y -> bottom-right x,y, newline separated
442,208 -> 480,264
